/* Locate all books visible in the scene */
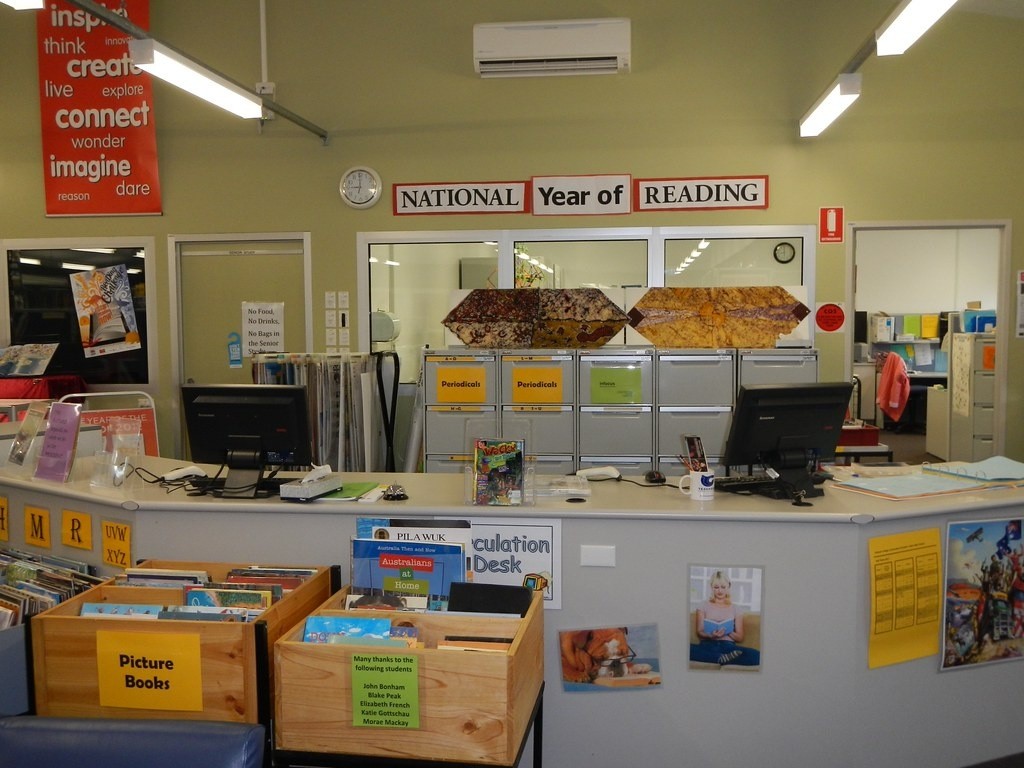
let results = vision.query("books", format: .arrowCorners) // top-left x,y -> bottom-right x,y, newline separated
831,456 -> 1024,501
304,581 -> 530,654
703,617 -> 734,637
0,547 -> 105,633
317,484 -> 388,503
473,436 -> 525,506
81,567 -> 318,623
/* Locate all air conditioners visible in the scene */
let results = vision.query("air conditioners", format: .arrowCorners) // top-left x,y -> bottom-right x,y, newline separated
472,17 -> 634,78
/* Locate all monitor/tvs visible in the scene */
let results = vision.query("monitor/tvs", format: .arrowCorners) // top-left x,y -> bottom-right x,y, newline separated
724,382 -> 854,498
182,384 -> 330,498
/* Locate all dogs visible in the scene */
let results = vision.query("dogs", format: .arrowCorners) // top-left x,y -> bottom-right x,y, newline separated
559,627 -> 652,684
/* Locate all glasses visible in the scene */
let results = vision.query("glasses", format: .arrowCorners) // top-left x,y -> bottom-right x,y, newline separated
584,647 -> 637,666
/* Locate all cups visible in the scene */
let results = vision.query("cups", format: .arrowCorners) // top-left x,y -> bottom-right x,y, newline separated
679,469 -> 715,500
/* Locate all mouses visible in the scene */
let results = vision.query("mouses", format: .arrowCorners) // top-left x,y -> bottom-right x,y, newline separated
645,470 -> 666,482
160,466 -> 208,481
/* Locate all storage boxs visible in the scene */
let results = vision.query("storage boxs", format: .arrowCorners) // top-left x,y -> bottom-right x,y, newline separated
31,560 -> 333,721
274,583 -> 543,765
0,550 -> 104,718
839,424 -> 880,446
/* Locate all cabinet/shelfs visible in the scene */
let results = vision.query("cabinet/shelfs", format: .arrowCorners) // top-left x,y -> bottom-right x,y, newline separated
421,346 -> 819,481
853,310 -> 949,425
926,309 -> 998,463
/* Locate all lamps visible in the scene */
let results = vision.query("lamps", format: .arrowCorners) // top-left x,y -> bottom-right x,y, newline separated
126,269 -> 143,274
61,262 -> 96,271
71,247 -> 117,254
876,0 -> 958,56
0,0 -> 44,10
19,258 -> 42,264
129,39 -> 263,120
799,72 -> 862,137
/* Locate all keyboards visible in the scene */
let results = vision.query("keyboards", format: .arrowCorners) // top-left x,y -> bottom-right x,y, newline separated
189,477 -> 303,489
714,475 -> 826,492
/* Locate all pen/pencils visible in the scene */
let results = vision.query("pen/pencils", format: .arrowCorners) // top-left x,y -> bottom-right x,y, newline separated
678,455 -> 694,471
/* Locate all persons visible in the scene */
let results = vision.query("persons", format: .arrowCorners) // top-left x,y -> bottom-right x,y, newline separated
690,572 -> 761,666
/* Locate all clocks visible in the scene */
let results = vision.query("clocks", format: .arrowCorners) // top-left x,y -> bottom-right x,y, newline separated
339,166 -> 382,209
774,242 -> 795,263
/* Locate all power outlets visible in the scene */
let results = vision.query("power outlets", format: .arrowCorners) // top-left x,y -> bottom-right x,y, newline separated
256,82 -> 275,95
